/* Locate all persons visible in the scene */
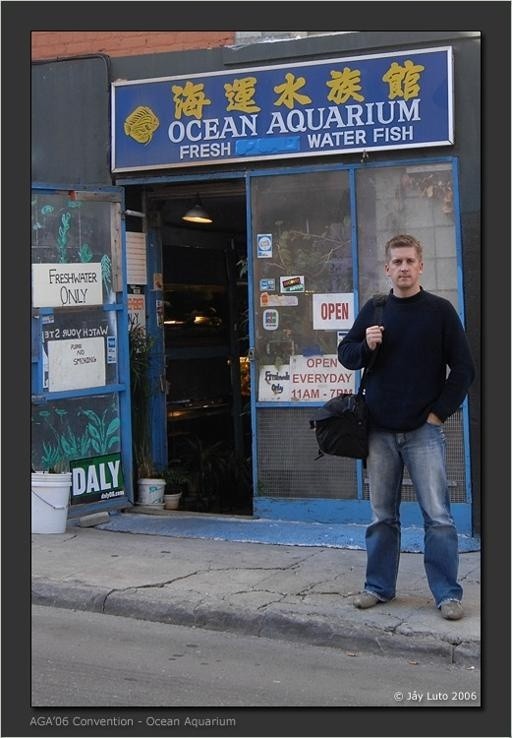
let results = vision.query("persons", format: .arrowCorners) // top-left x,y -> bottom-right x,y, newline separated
335,236 -> 477,620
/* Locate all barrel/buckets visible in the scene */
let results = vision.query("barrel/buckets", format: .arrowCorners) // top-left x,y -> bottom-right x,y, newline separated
136,477 -> 167,510
30,470 -> 72,534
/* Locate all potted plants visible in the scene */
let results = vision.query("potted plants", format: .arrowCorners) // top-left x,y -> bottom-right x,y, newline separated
161,472 -> 187,509
129,313 -> 166,511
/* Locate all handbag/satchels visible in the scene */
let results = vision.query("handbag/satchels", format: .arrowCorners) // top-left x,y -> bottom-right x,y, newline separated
306,378 -> 370,461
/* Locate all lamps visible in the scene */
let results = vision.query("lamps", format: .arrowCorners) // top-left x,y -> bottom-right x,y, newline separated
181,193 -> 214,224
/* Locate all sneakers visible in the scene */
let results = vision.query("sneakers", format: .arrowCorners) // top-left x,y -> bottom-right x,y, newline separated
352,587 -> 380,608
440,600 -> 465,620
